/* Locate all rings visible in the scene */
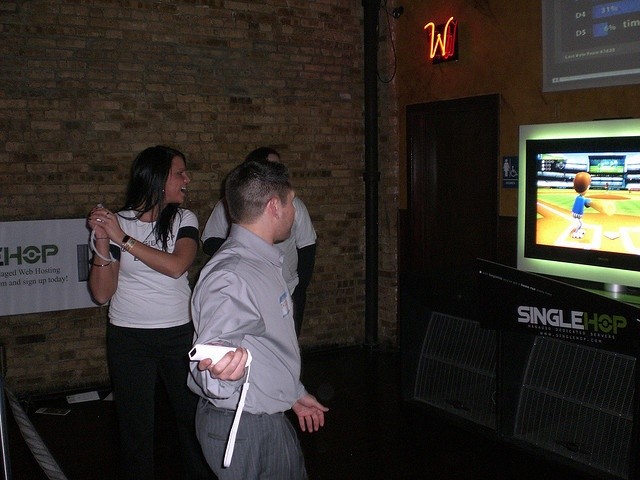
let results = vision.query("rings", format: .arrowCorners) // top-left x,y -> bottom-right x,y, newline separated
107,211 -> 109,218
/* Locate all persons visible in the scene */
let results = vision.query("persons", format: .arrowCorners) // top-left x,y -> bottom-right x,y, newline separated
202,146 -> 318,339
571,172 -> 604,239
186,158 -> 329,479
85,145 -> 201,479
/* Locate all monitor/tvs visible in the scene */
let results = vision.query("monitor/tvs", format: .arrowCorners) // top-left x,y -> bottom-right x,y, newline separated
517,118 -> 640,304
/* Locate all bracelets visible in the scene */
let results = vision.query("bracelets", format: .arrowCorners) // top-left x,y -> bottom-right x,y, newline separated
92,262 -> 112,267
123,237 -> 136,251
